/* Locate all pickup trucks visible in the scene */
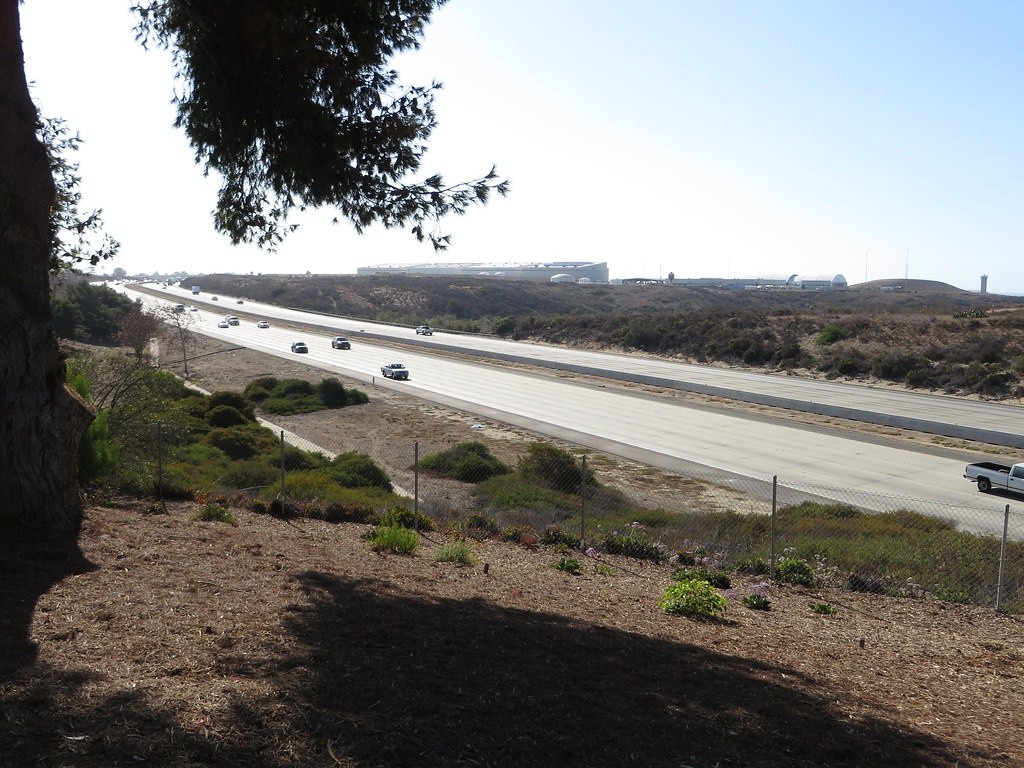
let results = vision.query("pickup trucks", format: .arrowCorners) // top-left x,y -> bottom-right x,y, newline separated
963,461 -> 1024,499
415,326 -> 434,336
380,364 -> 409,381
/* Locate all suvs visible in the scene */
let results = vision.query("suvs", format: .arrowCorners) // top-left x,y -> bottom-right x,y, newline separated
333,337 -> 351,350
174,304 -> 185,314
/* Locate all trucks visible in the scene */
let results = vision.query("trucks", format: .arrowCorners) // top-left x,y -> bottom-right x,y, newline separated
192,286 -> 202,296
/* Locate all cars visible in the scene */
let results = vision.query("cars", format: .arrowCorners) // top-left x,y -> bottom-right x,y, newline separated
218,321 -> 229,328
290,341 -> 308,354
97,272 -> 189,293
189,305 -> 198,311
228,318 -> 240,326
237,299 -> 243,305
224,315 -> 232,322
211,296 -> 219,301
257,321 -> 269,328
